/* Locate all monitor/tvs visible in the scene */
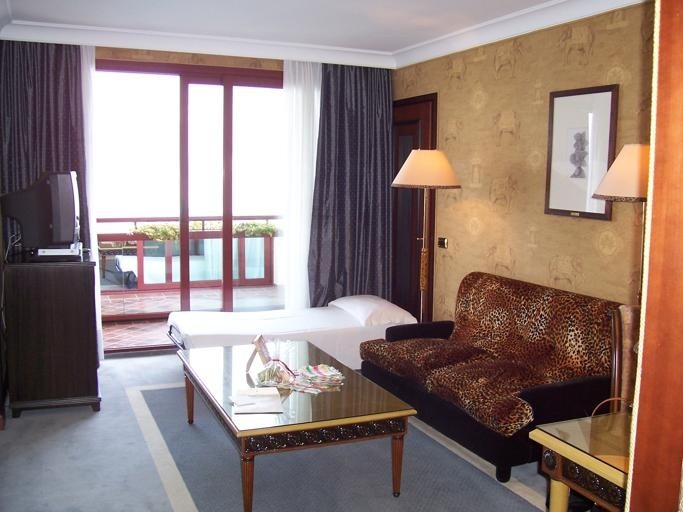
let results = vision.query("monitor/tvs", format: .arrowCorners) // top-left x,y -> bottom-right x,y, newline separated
0,171 -> 80,248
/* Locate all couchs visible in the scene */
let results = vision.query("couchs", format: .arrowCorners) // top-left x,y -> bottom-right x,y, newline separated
360,270 -> 630,483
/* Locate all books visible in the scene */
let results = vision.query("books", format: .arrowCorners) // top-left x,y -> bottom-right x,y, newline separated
28,255 -> 81,263
230,386 -> 284,415
37,247 -> 80,257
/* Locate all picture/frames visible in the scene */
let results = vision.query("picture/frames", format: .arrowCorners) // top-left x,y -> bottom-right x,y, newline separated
543,82 -> 616,219
245,334 -> 271,372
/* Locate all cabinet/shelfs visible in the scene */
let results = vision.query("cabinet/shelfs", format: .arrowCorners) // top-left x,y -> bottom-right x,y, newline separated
0,250 -> 101,418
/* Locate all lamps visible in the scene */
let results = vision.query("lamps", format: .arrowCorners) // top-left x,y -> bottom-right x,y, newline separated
591,144 -> 650,307
391,149 -> 463,323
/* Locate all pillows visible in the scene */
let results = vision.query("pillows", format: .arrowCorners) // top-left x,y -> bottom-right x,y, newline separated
331,294 -> 411,328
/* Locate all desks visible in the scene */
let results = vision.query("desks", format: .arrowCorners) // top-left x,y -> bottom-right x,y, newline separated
529,410 -> 637,511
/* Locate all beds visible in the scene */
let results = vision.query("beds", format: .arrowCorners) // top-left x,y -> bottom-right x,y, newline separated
166,292 -> 419,372
